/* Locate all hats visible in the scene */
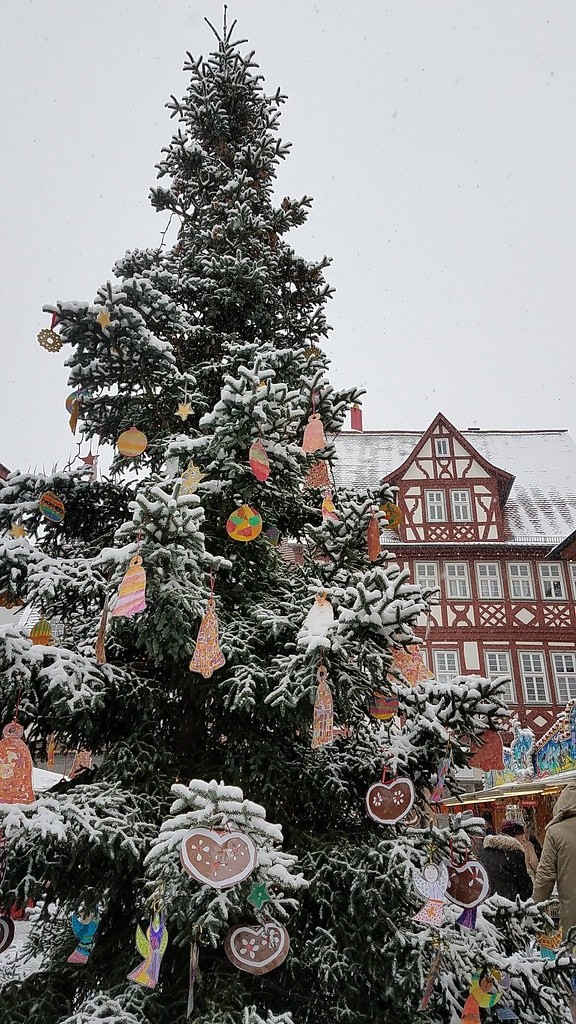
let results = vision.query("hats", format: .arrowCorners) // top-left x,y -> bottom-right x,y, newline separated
501,804 -> 527,829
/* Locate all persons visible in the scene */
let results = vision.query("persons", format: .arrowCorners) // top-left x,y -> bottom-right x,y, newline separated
531,781 -> 576,1024
475,818 -> 534,903
458,803 -> 543,866
